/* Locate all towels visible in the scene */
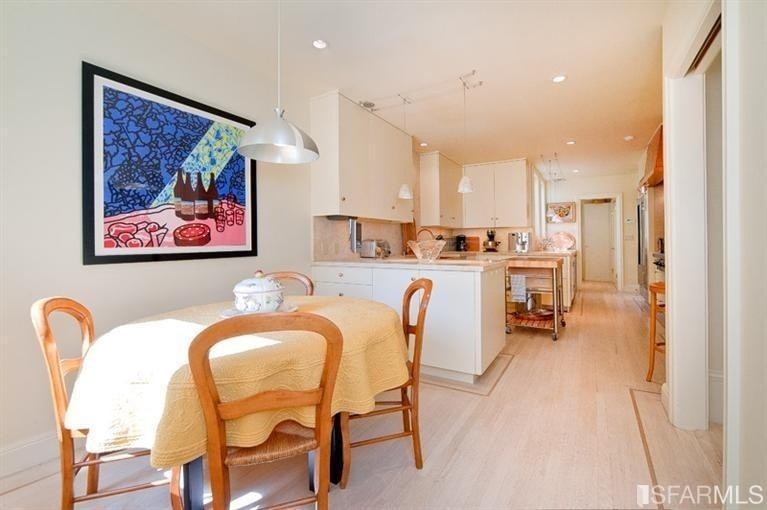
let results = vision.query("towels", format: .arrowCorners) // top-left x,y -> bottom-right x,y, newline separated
511,275 -> 527,302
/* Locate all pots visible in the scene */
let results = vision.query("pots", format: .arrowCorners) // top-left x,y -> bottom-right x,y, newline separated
234,270 -> 284,312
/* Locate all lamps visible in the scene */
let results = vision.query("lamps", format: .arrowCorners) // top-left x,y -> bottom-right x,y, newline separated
236,0 -> 319,165
399,98 -> 413,199
457,82 -> 473,194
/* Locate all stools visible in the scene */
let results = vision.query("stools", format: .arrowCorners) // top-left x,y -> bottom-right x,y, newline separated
646,282 -> 665,382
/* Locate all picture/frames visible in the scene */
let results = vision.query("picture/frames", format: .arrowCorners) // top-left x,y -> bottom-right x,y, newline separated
81,60 -> 258,266
545,201 -> 575,224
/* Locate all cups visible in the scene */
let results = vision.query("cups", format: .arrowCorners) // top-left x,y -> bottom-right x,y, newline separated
228,194 -> 233,204
214,207 -> 224,222
226,209 -> 234,226
236,209 -> 243,225
217,215 -> 224,232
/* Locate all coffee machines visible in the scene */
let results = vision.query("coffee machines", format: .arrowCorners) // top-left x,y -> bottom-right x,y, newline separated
455,235 -> 467,251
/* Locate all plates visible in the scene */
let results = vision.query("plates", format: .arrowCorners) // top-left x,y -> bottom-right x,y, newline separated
223,304 -> 297,318
549,232 -> 575,251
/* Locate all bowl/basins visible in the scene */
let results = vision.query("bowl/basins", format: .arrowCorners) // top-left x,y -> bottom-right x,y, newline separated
408,240 -> 447,263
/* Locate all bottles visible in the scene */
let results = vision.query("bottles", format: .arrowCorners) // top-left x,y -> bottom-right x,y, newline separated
174,168 -> 184,217
181,173 -> 196,221
221,198 -> 227,207
195,173 -> 209,220
207,173 -> 218,219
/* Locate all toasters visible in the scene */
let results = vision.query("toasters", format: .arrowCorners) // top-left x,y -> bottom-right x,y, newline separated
361,239 -> 391,257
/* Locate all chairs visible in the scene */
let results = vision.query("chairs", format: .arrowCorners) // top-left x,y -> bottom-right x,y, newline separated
169,311 -> 344,509
339,278 -> 432,490
29,296 -> 172,509
266,270 -> 313,296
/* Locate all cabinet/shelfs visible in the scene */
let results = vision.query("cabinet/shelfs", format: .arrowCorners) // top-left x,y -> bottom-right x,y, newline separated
371,268 -> 507,384
312,266 -> 371,296
312,92 -> 414,224
462,157 -> 532,229
420,150 -> 463,230
541,256 -> 576,312
